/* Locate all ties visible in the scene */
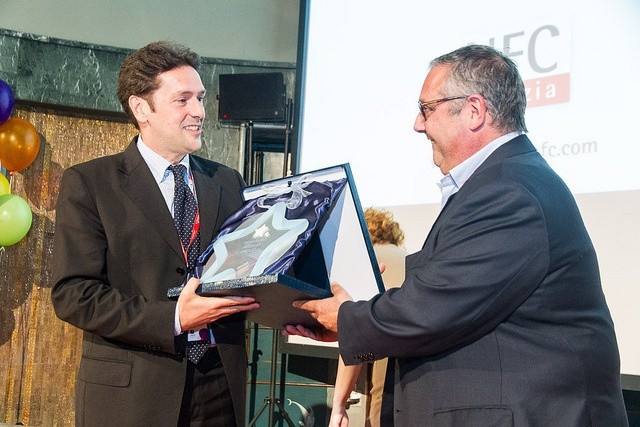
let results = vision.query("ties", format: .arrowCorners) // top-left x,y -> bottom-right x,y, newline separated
168,163 -> 212,364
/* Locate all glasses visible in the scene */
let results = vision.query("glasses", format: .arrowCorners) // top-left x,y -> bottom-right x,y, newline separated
417,94 -> 470,121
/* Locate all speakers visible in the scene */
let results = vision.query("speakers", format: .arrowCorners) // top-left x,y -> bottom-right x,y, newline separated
219,72 -> 285,121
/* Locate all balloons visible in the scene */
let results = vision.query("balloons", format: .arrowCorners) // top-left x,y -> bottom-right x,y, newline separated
0,79 -> 14,124
0,118 -> 41,176
0,173 -> 10,194
0,194 -> 32,249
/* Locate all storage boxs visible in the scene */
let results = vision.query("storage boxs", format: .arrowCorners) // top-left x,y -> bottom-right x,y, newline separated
168,162 -> 386,328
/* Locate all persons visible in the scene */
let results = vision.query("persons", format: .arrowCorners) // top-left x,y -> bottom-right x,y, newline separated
282,45 -> 630,427
326,207 -> 410,427
50,41 -> 259,426
